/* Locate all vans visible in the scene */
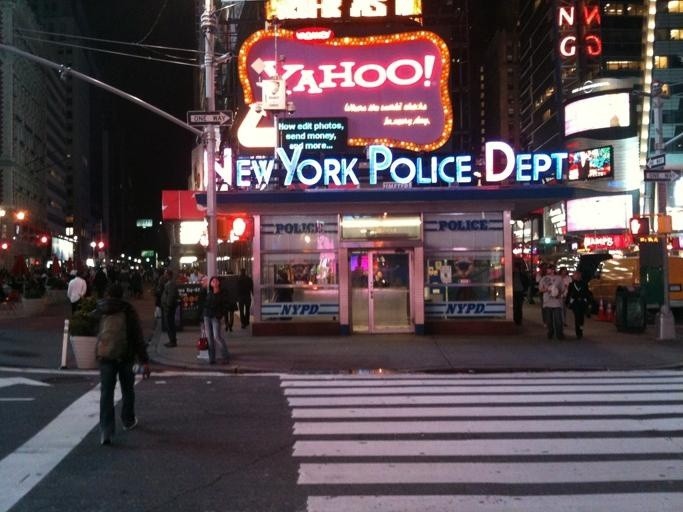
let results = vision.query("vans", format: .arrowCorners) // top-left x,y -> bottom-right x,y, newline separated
587,255 -> 683,323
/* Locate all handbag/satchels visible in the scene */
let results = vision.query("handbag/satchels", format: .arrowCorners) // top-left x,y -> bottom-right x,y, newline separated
568,296 -> 577,309
196,337 -> 208,350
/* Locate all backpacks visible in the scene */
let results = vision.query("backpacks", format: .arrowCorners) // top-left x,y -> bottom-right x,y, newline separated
94,309 -> 128,362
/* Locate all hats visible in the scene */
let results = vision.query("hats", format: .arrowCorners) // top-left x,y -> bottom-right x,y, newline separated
546,263 -> 557,269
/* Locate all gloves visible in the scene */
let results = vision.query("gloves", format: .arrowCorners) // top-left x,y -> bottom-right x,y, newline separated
142,362 -> 150,379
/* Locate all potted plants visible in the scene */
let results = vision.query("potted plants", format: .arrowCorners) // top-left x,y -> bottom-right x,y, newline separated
19,273 -> 51,318
68,296 -> 98,370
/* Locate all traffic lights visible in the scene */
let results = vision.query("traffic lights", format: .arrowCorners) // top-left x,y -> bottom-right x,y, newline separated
629,216 -> 650,239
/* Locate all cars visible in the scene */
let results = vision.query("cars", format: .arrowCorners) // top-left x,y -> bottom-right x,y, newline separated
525,254 -> 613,285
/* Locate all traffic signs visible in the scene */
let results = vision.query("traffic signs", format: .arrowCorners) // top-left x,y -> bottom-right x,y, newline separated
189,111 -> 233,126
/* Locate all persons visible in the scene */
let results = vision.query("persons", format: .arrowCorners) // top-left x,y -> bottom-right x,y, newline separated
270,270 -> 294,303
510,256 -> 597,341
86,282 -> 151,445
0,254 -> 253,365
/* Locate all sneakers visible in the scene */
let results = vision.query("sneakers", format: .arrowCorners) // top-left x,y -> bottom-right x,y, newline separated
122,417 -> 137,431
101,435 -> 112,444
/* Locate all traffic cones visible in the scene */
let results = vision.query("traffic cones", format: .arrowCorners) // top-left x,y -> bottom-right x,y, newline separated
594,296 -> 614,323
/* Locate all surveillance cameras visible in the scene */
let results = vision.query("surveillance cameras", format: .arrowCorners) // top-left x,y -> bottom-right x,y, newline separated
287,105 -> 296,115
256,105 -> 262,113
